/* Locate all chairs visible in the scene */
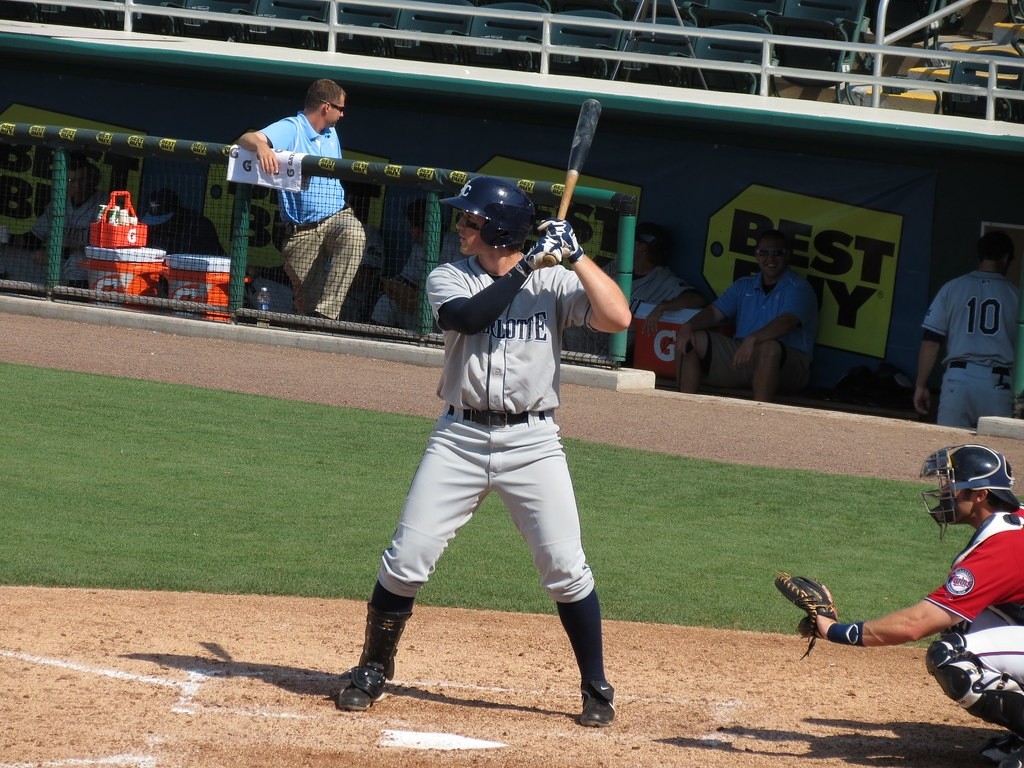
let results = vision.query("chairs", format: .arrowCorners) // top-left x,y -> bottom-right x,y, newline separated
0,0 -> 1024,127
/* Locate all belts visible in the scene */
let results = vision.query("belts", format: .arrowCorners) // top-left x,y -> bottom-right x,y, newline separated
949,361 -> 1010,376
291,203 -> 349,233
448,405 -> 545,427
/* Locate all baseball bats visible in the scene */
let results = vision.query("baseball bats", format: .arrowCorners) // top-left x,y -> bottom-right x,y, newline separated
541,98 -> 602,268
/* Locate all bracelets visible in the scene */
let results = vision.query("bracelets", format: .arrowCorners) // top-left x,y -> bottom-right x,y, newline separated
827,622 -> 865,647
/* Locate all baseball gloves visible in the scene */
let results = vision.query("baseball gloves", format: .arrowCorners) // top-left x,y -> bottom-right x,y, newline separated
775,569 -> 839,661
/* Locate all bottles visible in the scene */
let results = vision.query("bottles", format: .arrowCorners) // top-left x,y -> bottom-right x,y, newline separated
257,287 -> 270,328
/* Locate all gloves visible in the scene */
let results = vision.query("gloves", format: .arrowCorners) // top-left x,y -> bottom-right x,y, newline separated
537,217 -> 584,264
523,235 -> 573,270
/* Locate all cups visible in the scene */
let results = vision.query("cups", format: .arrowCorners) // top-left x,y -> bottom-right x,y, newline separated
95,204 -> 138,225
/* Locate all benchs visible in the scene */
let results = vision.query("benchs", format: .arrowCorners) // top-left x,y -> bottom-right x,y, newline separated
655,378 -> 938,424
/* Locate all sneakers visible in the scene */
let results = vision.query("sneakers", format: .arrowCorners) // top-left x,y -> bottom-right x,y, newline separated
580,683 -> 615,726
338,680 -> 372,711
980,733 -> 1024,761
997,754 -> 1024,768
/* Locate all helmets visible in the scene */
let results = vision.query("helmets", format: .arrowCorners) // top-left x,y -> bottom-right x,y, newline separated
439,176 -> 535,247
635,221 -> 673,254
942,443 -> 1020,509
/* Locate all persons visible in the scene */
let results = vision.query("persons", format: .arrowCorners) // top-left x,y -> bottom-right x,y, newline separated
561,222 -> 707,368
774,445 -> 1024,768
143,188 -> 227,299
676,229 -> 818,402
914,230 -> 1019,429
338,176 -> 632,727
239,79 -> 367,331
9,154 -> 110,304
254,196 -> 385,324
370,198 -> 473,335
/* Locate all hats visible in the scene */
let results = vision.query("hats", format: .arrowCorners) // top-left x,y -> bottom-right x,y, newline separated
143,187 -> 178,225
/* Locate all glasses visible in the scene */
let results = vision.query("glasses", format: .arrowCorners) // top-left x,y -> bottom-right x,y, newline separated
320,99 -> 345,113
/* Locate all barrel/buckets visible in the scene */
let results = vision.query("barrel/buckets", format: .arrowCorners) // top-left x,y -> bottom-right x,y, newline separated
165,253 -> 232,321
78,247 -> 166,309
